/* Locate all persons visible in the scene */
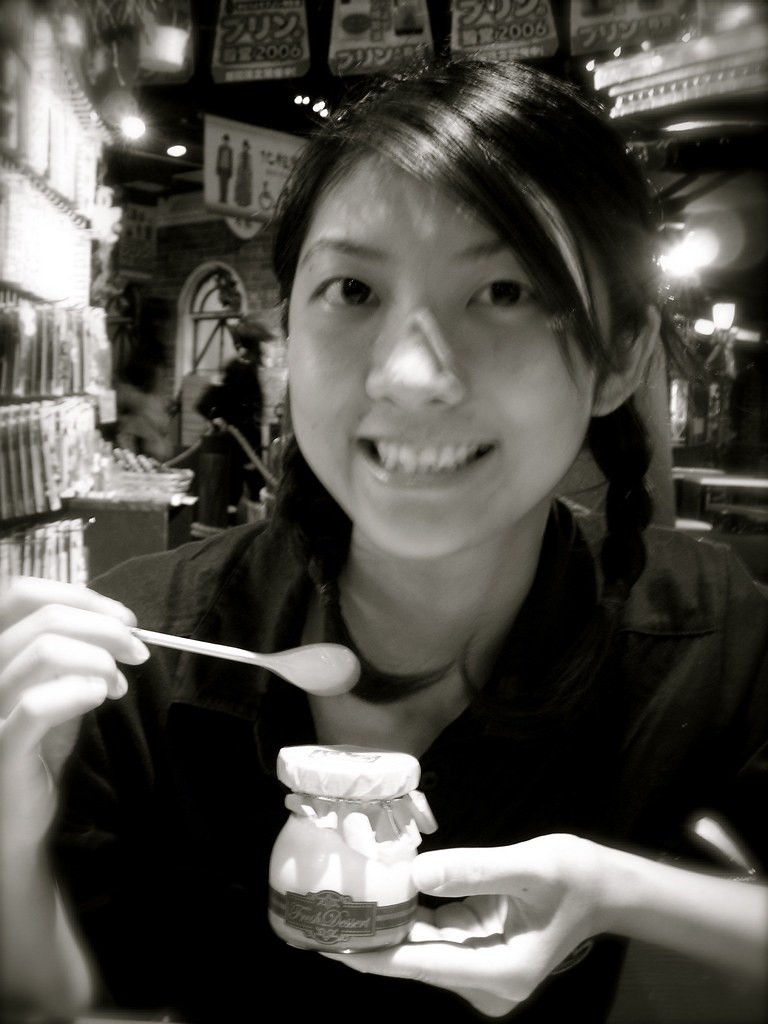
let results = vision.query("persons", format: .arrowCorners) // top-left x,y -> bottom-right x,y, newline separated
0,58 -> 768,1023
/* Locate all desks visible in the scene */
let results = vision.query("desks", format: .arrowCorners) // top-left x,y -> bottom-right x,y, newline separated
61,495 -> 197,579
672,466 -> 768,521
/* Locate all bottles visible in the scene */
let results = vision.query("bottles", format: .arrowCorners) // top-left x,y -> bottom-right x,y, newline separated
266,749 -> 421,953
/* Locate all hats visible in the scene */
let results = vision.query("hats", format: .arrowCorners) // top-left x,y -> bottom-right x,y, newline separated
229,324 -> 271,347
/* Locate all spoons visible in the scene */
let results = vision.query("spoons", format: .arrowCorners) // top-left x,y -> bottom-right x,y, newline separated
128,626 -> 362,697
